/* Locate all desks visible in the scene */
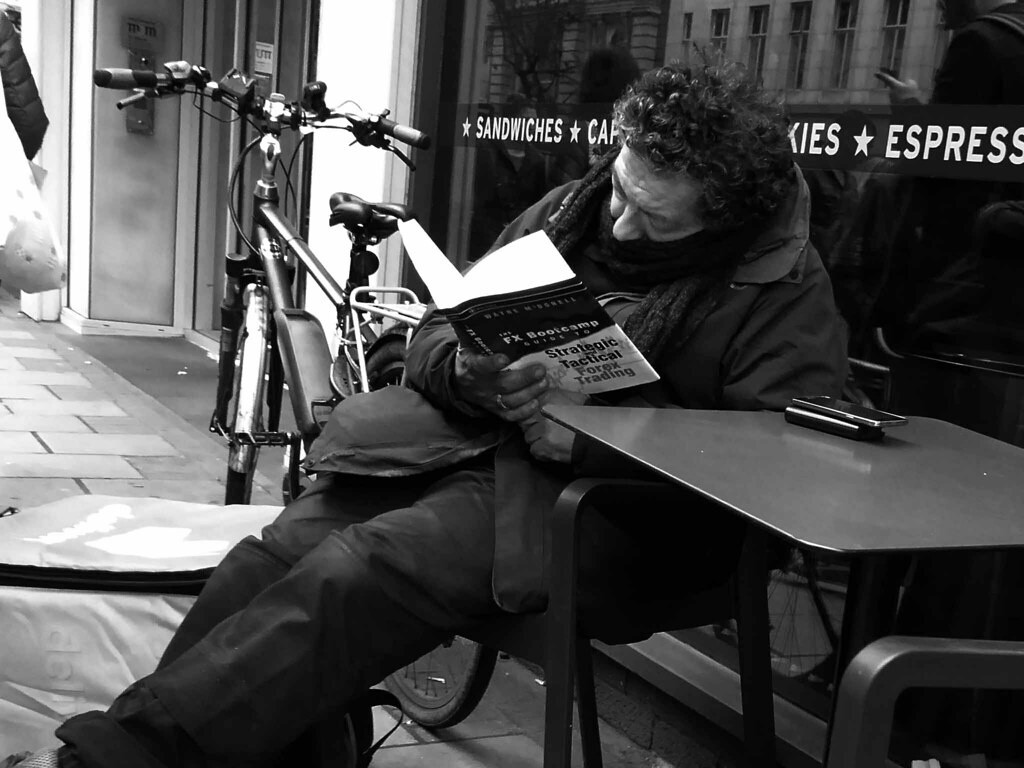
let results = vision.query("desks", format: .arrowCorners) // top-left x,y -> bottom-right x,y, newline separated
542,402 -> 1024,768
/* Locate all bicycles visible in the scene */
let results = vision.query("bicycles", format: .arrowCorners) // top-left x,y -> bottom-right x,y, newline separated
93,57 -> 498,728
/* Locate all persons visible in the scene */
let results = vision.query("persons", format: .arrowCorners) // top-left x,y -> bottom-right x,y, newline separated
797,0 -> 1024,362
466,91 -> 599,250
52,65 -> 861,768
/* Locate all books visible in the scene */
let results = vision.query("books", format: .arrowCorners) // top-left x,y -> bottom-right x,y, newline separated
395,213 -> 661,397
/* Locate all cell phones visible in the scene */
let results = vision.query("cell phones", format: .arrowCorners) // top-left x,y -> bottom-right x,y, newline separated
792,396 -> 908,427
